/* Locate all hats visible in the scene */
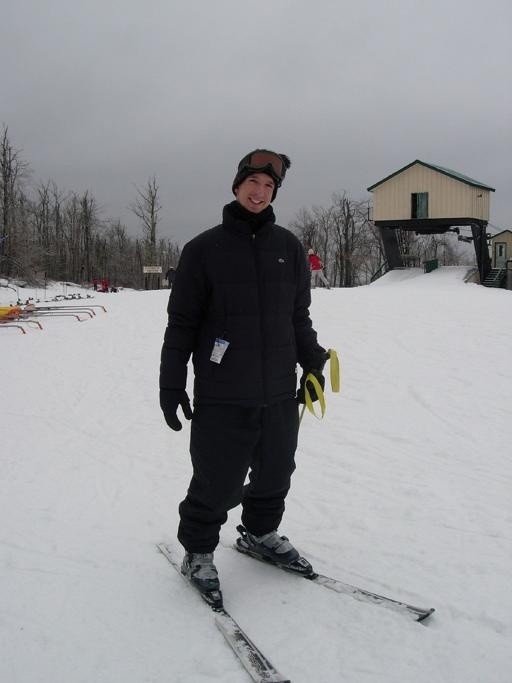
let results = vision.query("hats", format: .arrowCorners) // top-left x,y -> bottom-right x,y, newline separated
308,248 -> 314,255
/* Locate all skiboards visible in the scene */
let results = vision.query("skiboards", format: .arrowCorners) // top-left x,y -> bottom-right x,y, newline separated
158,534 -> 435,683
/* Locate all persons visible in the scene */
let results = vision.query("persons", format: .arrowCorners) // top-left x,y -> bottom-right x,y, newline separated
165,266 -> 176,288
306,248 -> 330,289
160,150 -> 330,589
92,275 -> 118,293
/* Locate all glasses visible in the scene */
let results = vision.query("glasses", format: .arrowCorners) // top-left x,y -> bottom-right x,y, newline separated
239,151 -> 283,177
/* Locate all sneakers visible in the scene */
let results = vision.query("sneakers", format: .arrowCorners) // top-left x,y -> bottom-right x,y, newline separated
243,529 -> 299,565
231,150 -> 290,203
180,550 -> 220,591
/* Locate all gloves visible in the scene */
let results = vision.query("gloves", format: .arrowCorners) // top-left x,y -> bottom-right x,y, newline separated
160,387 -> 192,431
297,372 -> 325,404
